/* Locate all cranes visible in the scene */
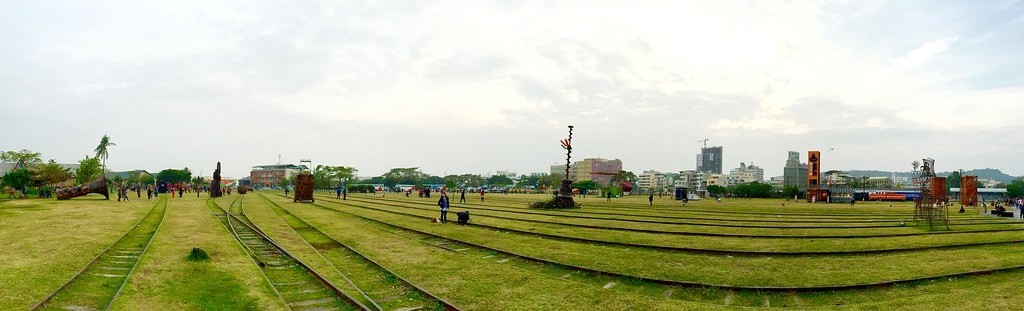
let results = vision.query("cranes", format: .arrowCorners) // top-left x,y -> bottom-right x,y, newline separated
697,138 -> 709,147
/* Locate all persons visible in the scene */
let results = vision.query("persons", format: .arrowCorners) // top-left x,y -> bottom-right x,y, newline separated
361,185 -> 447,198
606,191 -> 613,201
7,183 -> 62,199
958,206 -> 965,213
285,188 -> 289,195
438,192 -> 450,223
794,191 -> 856,205
966,197 -> 1024,219
889,200 -> 893,207
337,187 -> 341,200
460,189 -> 466,204
343,186 -> 347,200
481,189 -> 485,202
649,193 -> 653,206
657,191 -> 753,207
457,210 -> 470,227
114,182 -> 254,203
